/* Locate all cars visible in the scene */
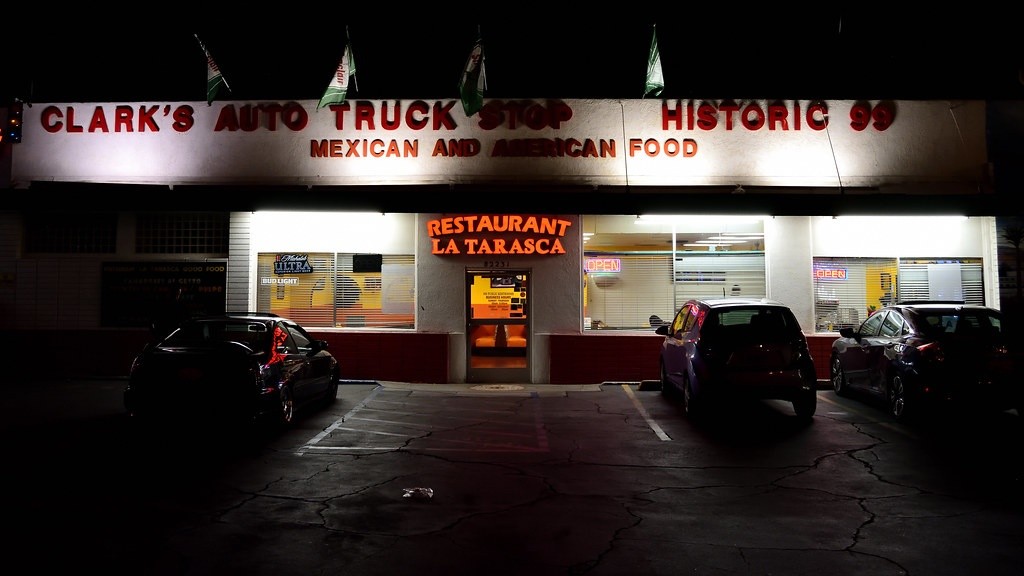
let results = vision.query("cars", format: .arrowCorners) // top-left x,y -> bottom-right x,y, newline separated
123,312 -> 339,436
655,300 -> 816,425
829,300 -> 1024,433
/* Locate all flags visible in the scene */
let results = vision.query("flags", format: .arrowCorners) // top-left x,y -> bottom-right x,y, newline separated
204,49 -> 223,108
316,44 -> 356,111
457,37 -> 485,118
642,29 -> 664,99
